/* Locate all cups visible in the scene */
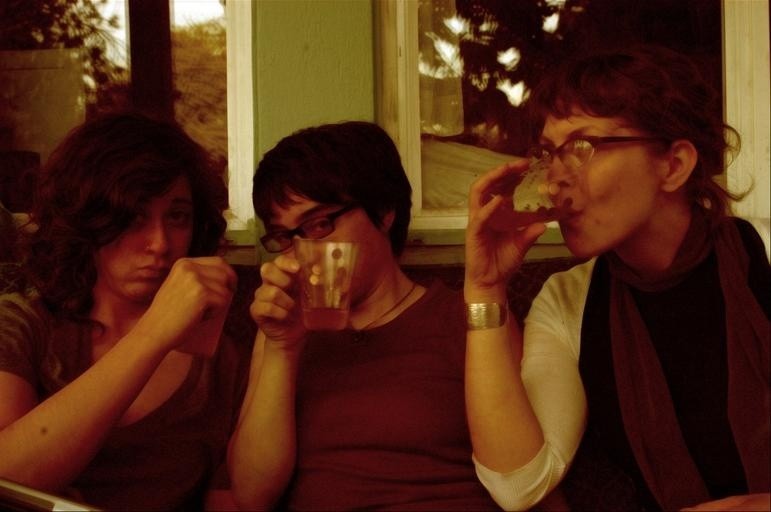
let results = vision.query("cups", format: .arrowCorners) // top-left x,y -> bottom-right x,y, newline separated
482,153 -> 585,232
172,296 -> 231,357
295,241 -> 354,332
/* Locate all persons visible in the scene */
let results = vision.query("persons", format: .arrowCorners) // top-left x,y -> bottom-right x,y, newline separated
0,107 -> 240,512
461,44 -> 770,512
227,119 -> 520,512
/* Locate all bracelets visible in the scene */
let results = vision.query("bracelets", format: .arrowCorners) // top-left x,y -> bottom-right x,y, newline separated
460,297 -> 514,332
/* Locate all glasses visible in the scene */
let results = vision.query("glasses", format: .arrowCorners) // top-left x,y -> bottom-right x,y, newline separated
258,198 -> 364,254
525,131 -> 672,168
